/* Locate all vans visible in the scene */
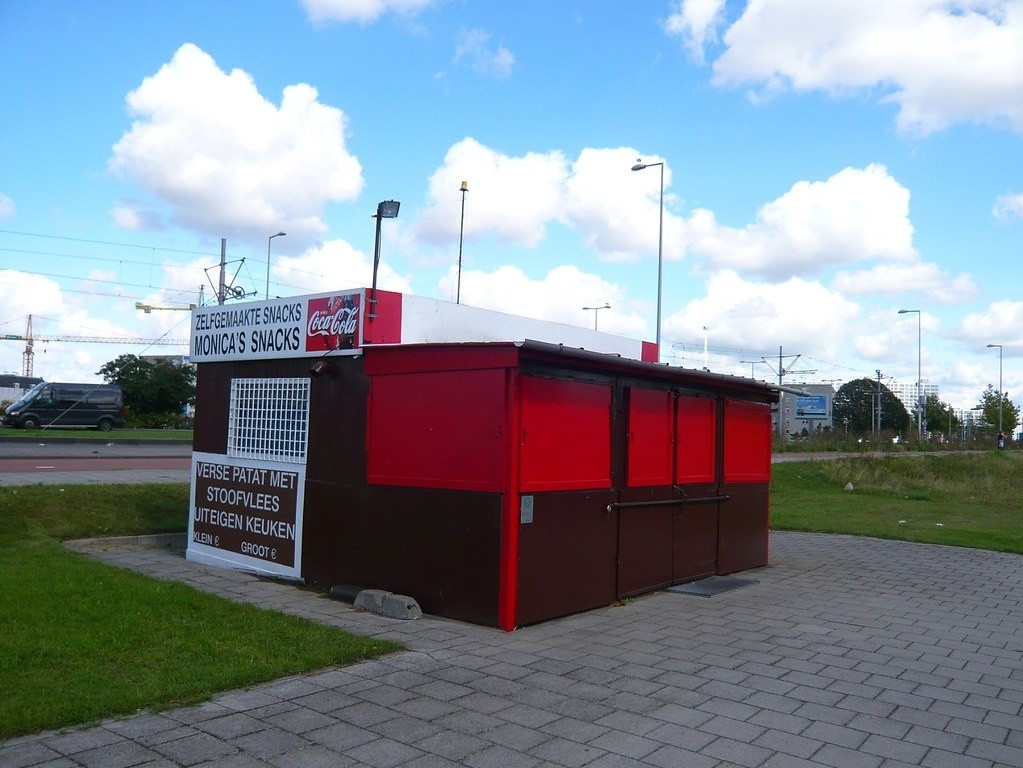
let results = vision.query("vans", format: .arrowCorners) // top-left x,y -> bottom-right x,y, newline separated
1,381 -> 125,432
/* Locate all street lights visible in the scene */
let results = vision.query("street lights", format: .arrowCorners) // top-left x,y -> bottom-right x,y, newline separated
898,309 -> 921,442
632,162 -> 663,361
266,233 -> 287,300
987,344 -> 1002,433
583,305 -> 610,332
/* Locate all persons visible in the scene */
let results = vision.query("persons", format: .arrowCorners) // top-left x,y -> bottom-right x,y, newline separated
998,430 -> 1005,451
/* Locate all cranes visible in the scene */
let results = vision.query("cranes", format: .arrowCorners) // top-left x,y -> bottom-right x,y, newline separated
0,313 -> 191,378
